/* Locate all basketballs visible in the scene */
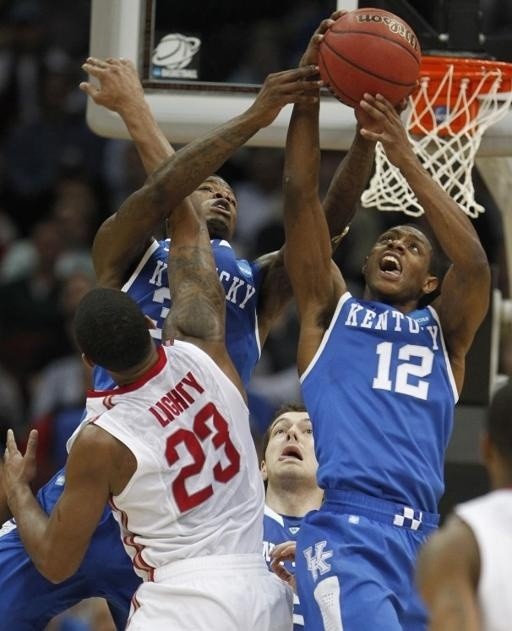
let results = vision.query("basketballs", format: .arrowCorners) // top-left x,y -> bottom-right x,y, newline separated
319,9 -> 420,109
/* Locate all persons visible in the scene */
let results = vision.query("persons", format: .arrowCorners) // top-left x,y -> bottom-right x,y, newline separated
0,1 -> 512,631
